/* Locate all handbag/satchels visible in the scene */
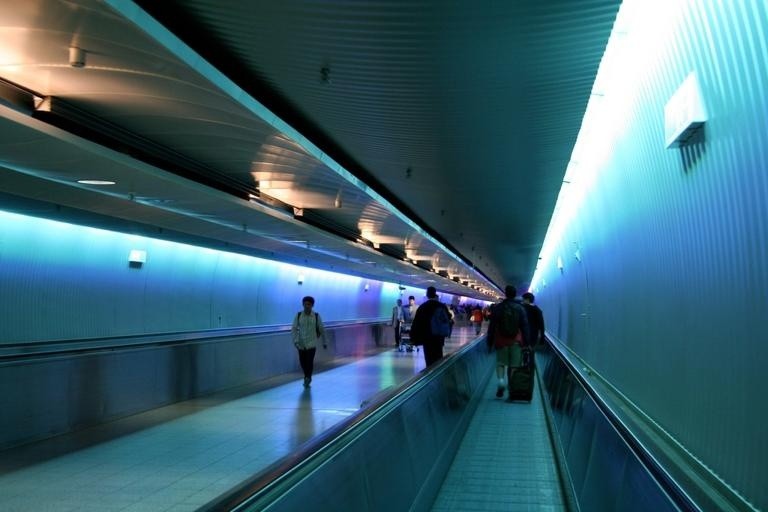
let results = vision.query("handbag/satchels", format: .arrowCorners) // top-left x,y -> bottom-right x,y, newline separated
432,308 -> 451,337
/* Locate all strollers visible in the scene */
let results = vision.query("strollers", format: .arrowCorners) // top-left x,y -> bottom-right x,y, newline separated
398,318 -> 420,353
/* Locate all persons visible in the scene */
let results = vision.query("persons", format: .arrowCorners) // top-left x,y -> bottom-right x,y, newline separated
446,299 -> 493,337
519,292 -> 546,366
407,285 -> 452,366
486,282 -> 529,399
389,299 -> 404,347
290,294 -> 328,390
398,296 -> 420,353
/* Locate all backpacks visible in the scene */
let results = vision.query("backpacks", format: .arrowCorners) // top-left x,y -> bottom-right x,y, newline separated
496,300 -> 518,338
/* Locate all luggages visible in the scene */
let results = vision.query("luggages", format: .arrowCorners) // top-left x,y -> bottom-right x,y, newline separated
509,349 -> 534,403
